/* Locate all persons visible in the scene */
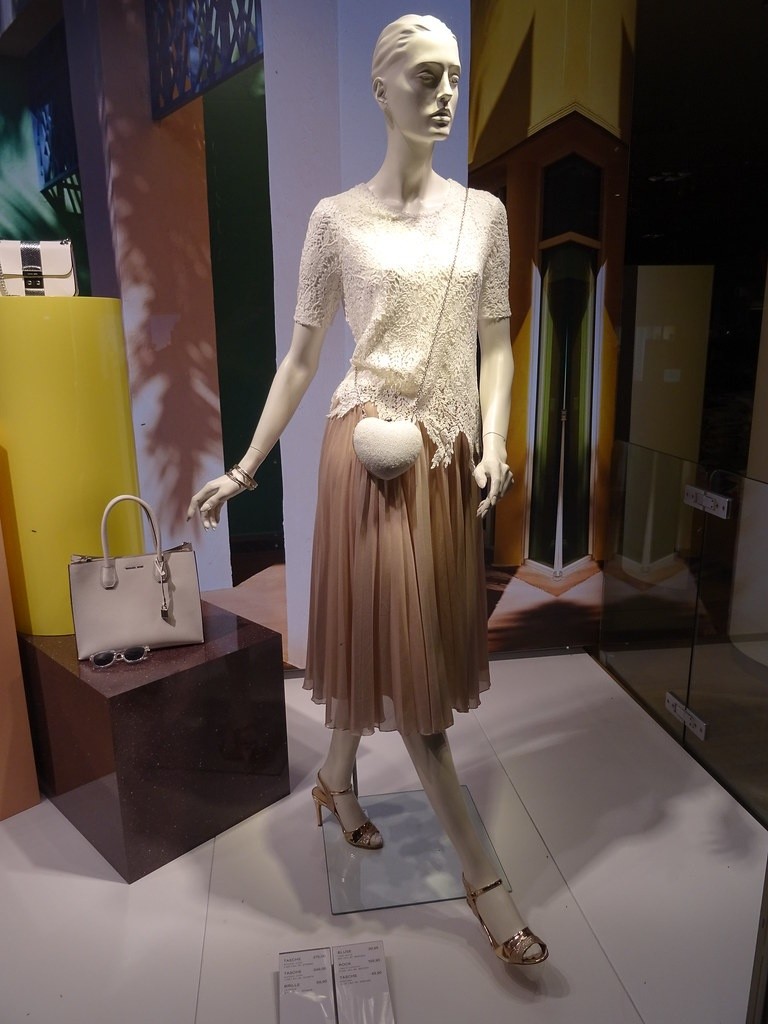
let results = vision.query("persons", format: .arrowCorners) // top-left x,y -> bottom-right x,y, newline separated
187,13 -> 551,965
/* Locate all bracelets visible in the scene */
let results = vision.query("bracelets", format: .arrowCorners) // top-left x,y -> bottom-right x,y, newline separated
227,463 -> 257,491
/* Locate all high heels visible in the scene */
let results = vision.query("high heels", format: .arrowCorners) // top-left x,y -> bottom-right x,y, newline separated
312,768 -> 384,849
462,870 -> 548,964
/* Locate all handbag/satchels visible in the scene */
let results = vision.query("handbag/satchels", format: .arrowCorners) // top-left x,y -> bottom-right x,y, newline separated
353,417 -> 423,481
0,239 -> 79,297
68,495 -> 204,661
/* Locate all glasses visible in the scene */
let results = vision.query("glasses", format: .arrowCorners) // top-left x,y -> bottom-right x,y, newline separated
89,646 -> 156,671
